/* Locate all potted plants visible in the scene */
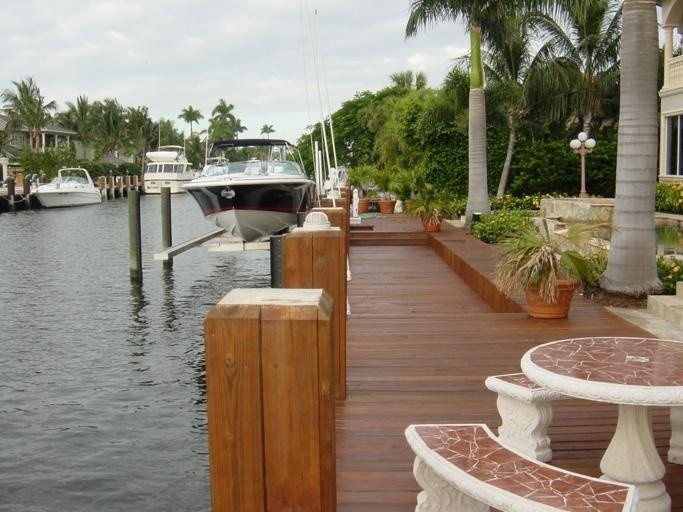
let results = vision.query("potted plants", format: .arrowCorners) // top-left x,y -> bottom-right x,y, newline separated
490,212 -> 621,318
346,163 -> 455,233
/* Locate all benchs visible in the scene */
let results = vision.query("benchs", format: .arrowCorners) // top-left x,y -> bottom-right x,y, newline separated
403,371 -> 683,511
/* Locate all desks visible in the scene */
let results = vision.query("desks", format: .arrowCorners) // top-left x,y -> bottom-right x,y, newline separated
519,337 -> 683,512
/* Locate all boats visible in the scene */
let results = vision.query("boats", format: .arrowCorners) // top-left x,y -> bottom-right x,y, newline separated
31,167 -> 102,210
143,143 -> 203,195
177,138 -> 317,243
